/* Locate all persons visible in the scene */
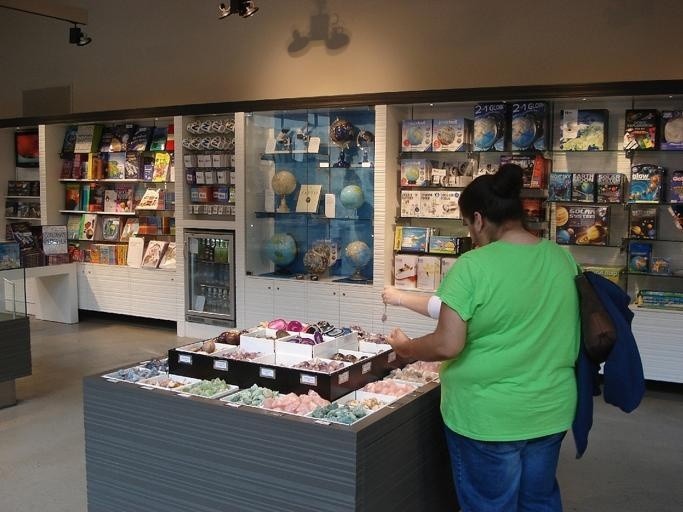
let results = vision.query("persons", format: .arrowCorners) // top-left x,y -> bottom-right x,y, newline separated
378,162 -> 584,511
380,282 -> 440,321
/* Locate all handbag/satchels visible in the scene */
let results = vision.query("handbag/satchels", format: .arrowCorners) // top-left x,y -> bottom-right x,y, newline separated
575,269 -> 618,363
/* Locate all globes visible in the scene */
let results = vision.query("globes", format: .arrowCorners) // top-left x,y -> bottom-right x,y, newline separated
270,171 -> 296,214
344,241 -> 372,281
339,185 -> 363,218
261,232 -> 298,275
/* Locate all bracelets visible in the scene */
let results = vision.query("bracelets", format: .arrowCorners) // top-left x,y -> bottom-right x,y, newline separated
398,293 -> 405,305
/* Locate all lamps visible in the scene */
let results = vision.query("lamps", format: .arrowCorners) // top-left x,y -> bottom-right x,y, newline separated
68,20 -> 91,48
219,1 -> 258,21
286,3 -> 351,58
